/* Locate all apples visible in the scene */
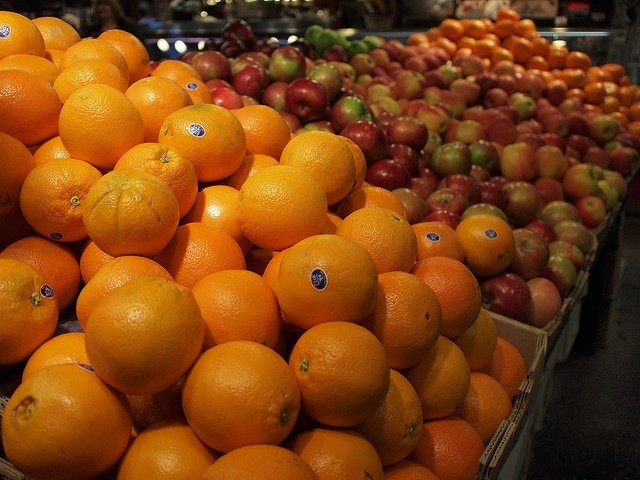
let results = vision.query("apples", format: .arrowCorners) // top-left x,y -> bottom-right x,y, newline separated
184,18 -> 627,327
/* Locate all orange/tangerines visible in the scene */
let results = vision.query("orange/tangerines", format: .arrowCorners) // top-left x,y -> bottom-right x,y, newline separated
0,11 -> 526,477
407,8 -> 640,135
